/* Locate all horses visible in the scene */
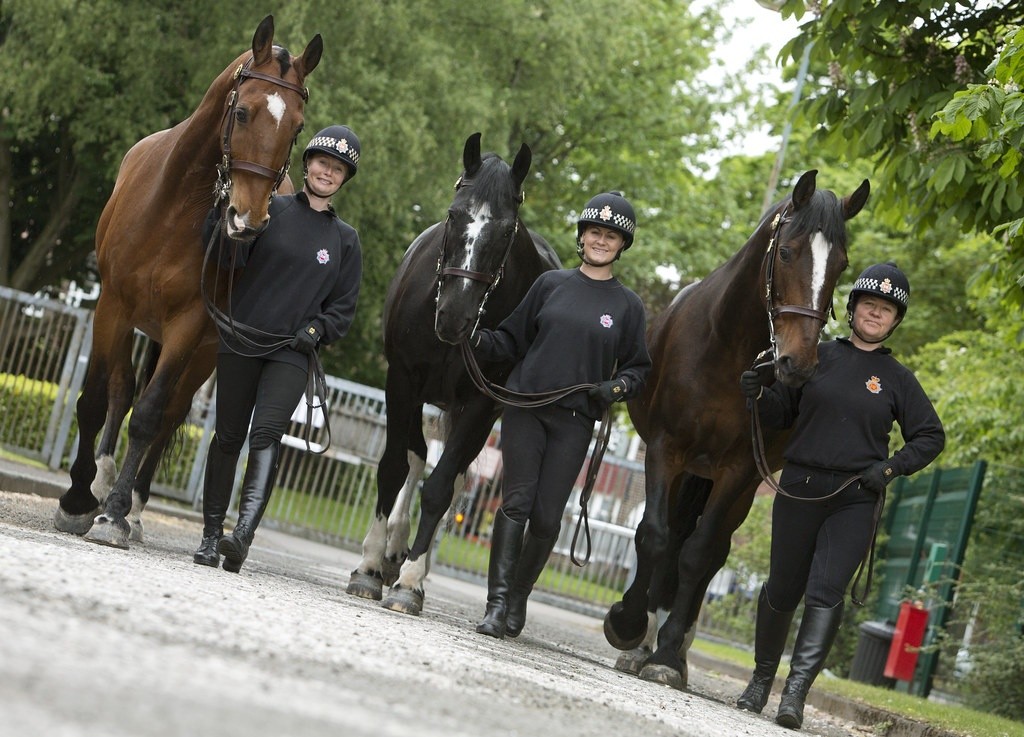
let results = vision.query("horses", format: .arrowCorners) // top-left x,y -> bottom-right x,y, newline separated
53,13 -> 325,551
603,169 -> 872,691
345,132 -> 564,617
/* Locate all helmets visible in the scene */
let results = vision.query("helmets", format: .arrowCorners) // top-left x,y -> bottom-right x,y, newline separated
577,190 -> 636,251
846,260 -> 910,320
303,125 -> 361,177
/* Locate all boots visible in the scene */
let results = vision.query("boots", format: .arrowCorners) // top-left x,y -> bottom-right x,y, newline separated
778,600 -> 845,731
194,434 -> 241,567
218,442 -> 281,573
738,582 -> 797,714
477,507 -> 526,639
506,524 -> 559,637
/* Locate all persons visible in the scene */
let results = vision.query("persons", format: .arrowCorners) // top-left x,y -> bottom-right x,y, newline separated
738,264 -> 946,728
193,123 -> 362,574
467,192 -> 651,638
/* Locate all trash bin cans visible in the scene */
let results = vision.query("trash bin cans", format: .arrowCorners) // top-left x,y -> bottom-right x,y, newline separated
849,618 -> 899,691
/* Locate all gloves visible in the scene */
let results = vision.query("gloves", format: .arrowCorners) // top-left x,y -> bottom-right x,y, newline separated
740,370 -> 764,400
290,320 -> 326,355
588,377 -> 624,407
862,459 -> 902,492
